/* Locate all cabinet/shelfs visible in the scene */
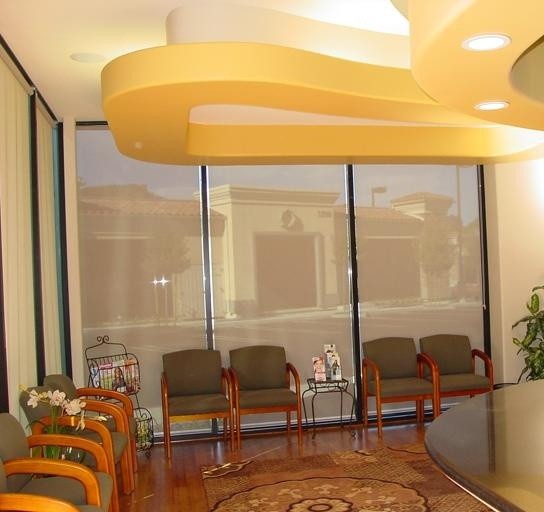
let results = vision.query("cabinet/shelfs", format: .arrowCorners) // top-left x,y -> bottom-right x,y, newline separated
85,334 -> 155,454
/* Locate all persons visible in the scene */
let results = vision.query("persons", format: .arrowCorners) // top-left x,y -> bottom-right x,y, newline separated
112,367 -> 126,392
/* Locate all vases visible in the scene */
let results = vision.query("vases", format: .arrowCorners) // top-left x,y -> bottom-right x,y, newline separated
42,426 -> 83,463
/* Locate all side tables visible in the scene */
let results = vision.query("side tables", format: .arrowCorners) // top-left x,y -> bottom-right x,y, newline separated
302,377 -> 356,439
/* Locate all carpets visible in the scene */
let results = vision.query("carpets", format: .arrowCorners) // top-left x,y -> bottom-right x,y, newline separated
200,444 -> 494,512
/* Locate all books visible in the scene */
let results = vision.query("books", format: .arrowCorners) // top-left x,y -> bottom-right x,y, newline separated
312,344 -> 342,382
90,359 -> 139,394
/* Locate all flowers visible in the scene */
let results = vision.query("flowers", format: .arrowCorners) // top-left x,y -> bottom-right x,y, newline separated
19,384 -> 107,458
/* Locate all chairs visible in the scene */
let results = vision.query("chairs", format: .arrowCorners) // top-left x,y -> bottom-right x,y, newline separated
0,413 -> 113,512
228,345 -> 302,450
161,349 -> 234,459
420,334 -> 493,416
19,386 -> 130,512
44,374 -> 137,491
0,457 -> 101,512
362,337 -> 438,438
